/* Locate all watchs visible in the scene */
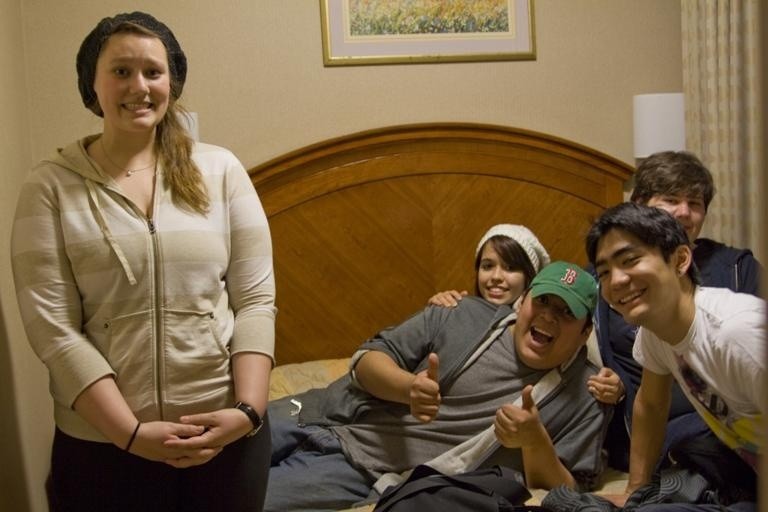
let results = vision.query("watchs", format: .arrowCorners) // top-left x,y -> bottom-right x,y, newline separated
234,399 -> 264,439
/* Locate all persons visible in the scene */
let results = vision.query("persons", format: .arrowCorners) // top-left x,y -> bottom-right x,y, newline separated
260,260 -> 620,510
584,202 -> 768,511
588,147 -> 768,502
6,11 -> 276,510
427,220 -> 627,409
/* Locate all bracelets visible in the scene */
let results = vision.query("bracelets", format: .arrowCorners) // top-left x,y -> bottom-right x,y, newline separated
124,421 -> 143,451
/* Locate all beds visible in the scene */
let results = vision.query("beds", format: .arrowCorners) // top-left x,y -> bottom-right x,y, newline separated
247,121 -> 638,512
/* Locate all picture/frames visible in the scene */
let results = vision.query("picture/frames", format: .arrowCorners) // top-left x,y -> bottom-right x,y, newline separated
319,0 -> 537,67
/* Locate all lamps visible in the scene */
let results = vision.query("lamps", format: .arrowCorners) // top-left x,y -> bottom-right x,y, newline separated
633,92 -> 686,159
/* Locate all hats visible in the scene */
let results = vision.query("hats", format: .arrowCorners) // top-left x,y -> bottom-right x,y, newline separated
528,259 -> 598,321
474,223 -> 551,275
76,10 -> 187,117
